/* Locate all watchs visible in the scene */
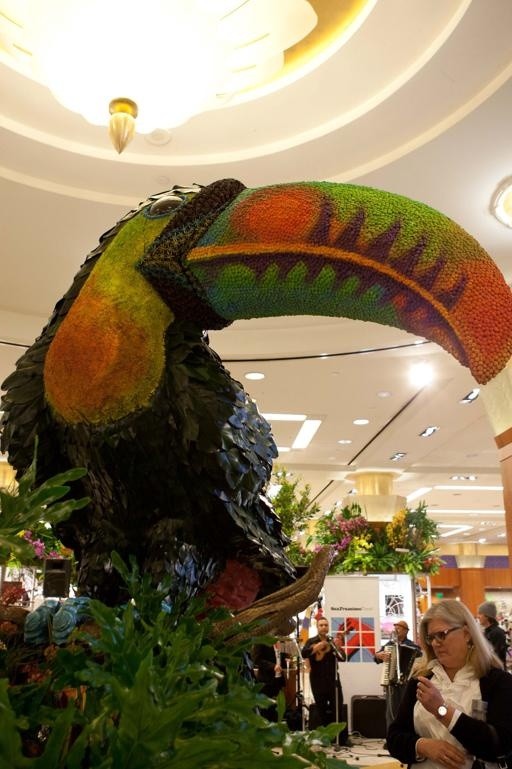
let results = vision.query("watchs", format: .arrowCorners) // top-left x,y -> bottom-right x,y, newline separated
435,700 -> 448,721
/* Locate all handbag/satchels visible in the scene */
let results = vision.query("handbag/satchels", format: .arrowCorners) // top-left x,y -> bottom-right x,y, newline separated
471,724 -> 509,769
351,694 -> 387,737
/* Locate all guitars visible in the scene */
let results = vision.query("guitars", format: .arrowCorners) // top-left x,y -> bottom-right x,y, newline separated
311,626 -> 354,660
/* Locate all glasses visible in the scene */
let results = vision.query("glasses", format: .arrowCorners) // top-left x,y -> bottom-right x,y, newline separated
424,627 -> 463,646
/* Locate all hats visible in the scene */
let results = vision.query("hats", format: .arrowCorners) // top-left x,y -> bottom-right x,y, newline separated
479,601 -> 497,619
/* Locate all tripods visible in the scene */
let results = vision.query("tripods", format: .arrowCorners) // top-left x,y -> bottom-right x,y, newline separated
377,643 -> 404,768
283,615 -> 309,730
323,640 -> 351,758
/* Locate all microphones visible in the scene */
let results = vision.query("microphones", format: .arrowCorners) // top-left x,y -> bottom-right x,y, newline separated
391,631 -> 396,641
325,633 -> 332,641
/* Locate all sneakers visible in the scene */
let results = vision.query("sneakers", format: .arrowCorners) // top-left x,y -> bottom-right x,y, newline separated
340,740 -> 352,746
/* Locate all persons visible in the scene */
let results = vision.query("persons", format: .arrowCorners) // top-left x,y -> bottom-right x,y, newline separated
387,599 -> 512,769
301,616 -> 352,748
371,619 -> 423,750
477,600 -> 505,669
253,637 -> 294,724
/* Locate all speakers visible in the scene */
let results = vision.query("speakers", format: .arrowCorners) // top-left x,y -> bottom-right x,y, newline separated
43,559 -> 72,597
309,703 -> 348,745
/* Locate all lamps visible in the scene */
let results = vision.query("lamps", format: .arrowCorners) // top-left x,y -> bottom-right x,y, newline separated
483,169 -> 512,238
3,4 -> 383,158
